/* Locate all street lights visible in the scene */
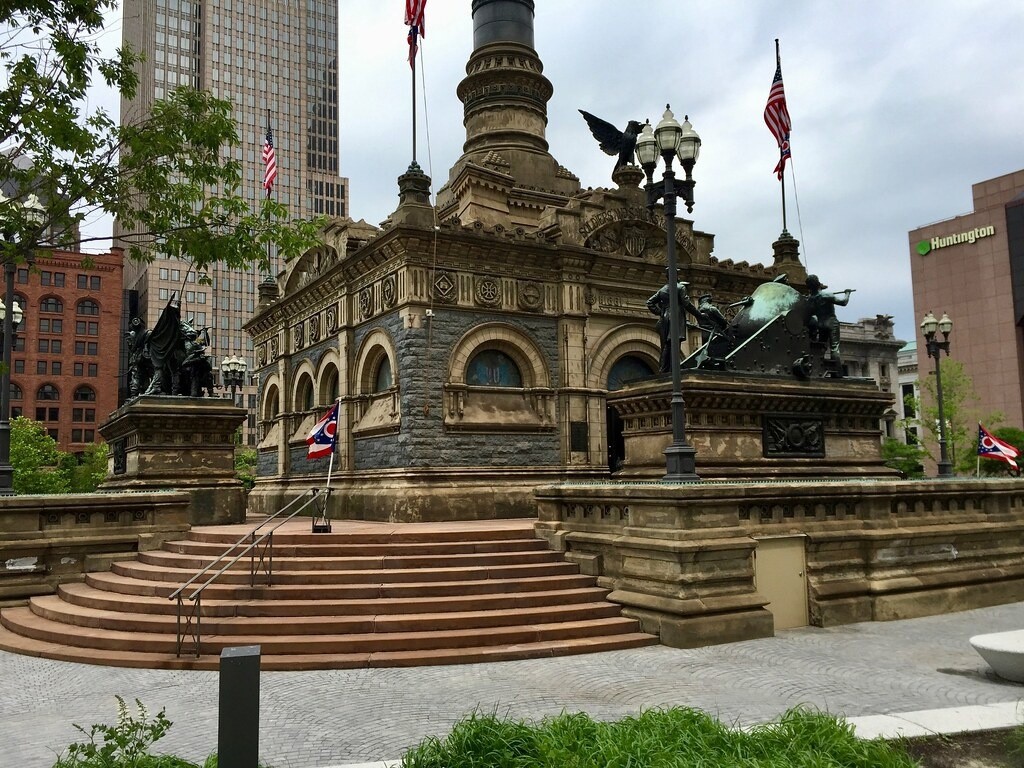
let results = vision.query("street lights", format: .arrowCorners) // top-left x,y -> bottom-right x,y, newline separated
919,310 -> 956,478
634,102 -> 703,487
0,299 -> 24,388
0,195 -> 46,495
221,353 -> 247,407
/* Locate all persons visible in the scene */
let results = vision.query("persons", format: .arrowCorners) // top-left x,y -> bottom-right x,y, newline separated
646,267 -> 701,374
125,319 -> 214,399
803,275 -> 851,377
698,294 -> 729,346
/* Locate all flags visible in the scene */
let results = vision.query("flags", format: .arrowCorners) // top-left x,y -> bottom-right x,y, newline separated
307,400 -> 337,457
403,0 -> 427,70
262,131 -> 276,190
762,51 -> 792,181
978,423 -> 1019,470
